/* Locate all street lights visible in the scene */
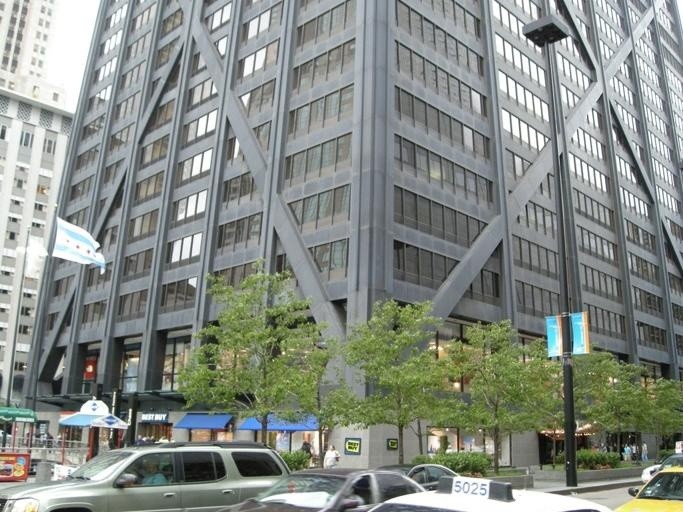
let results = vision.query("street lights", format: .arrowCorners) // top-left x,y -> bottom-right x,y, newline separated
522,16 -> 578,486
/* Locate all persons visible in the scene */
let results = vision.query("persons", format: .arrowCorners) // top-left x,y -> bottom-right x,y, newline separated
549,440 -> 650,464
0,429 -> 169,456
323,445 -> 340,468
300,440 -> 312,468
127,455 -> 167,485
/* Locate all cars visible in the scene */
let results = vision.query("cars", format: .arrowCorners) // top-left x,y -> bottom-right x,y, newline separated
372,462 -> 462,491
217,466 -> 430,512
365,474 -> 613,512
613,453 -> 683,512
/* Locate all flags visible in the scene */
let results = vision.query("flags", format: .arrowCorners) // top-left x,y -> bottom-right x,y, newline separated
51,218 -> 106,276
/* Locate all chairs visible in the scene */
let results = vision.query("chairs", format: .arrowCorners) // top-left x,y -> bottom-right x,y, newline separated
162,466 -> 172,483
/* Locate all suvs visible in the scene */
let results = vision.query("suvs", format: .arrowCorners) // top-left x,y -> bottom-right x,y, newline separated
1,441 -> 290,511
